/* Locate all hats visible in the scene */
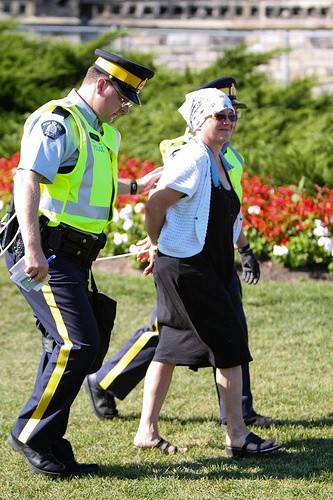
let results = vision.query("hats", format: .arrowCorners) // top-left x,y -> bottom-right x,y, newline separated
93,49 -> 155,105
197,77 -> 247,109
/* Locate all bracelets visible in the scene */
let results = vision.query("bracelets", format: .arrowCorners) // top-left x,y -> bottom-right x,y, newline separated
130,179 -> 137,195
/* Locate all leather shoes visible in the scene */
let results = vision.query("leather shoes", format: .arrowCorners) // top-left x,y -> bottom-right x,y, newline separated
6,432 -> 66,476
32,459 -> 102,479
83,372 -> 119,421
221,416 -> 281,428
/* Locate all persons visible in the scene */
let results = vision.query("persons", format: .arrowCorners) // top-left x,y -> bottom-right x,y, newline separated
0,49 -> 166,478
82,77 -> 274,428
133,87 -> 282,459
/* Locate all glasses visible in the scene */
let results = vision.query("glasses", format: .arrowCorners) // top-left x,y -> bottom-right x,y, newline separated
105,79 -> 133,107
207,113 -> 237,121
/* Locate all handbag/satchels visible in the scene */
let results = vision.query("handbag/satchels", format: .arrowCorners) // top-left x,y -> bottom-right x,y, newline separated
86,266 -> 117,374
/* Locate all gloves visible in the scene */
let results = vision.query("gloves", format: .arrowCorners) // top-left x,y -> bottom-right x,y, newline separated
239,242 -> 260,285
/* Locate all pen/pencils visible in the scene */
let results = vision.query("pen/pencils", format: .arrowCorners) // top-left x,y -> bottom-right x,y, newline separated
27,254 -> 56,282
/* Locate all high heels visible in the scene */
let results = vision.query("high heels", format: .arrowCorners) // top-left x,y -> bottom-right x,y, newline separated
225,432 -> 283,458
134,437 -> 177,455
